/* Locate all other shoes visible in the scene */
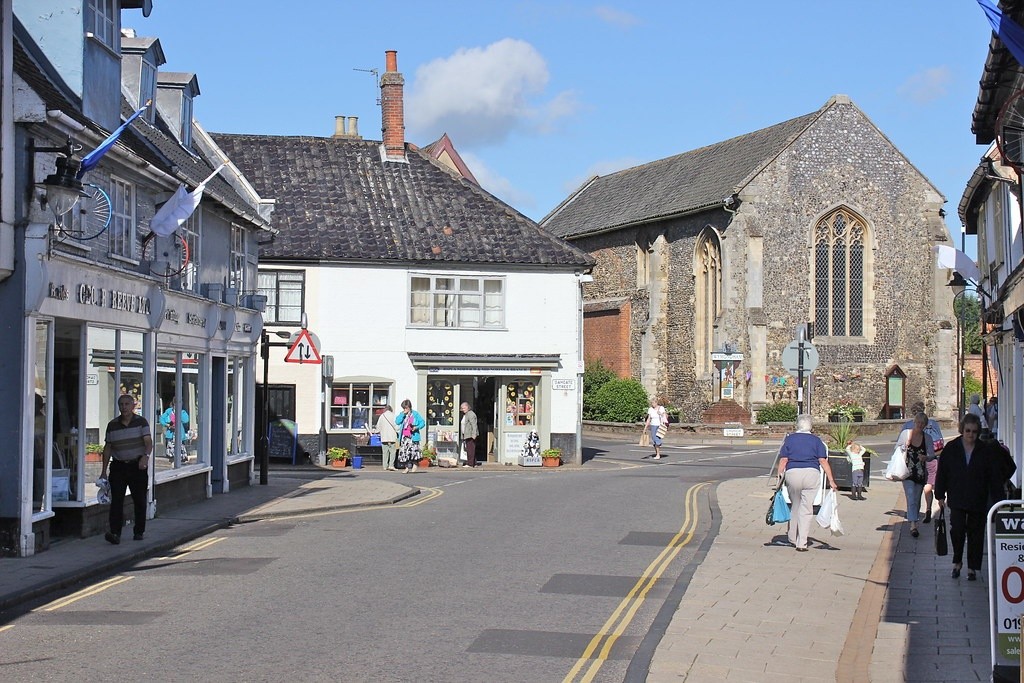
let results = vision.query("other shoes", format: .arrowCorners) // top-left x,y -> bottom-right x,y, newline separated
383,467 -> 389,470
654,455 -> 661,459
104,531 -> 121,544
922,509 -> 931,524
909,527 -> 919,537
133,530 -> 144,541
389,466 -> 398,471
850,495 -> 866,500
952,562 -> 963,578
463,464 -> 474,469
402,469 -> 409,473
787,539 -> 808,551
412,463 -> 419,472
968,569 -> 977,581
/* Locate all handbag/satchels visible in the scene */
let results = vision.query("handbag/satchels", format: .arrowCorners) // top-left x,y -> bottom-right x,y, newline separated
96,478 -> 111,505
816,490 -> 845,537
987,496 -> 997,522
932,440 -> 943,451
933,507 -> 948,555
396,433 -> 399,438
766,489 -> 792,526
884,447 -> 910,481
656,406 -> 669,439
639,432 -> 649,447
460,442 -> 469,461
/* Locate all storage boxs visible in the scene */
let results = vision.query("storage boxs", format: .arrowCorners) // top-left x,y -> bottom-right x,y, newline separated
351,432 -> 370,447
369,434 -> 382,446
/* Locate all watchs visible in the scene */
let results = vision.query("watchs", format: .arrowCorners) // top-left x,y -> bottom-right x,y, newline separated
144,454 -> 150,458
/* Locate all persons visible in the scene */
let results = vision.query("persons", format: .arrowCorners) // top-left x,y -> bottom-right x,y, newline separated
845,444 -> 867,500
395,399 -> 425,474
159,401 -> 189,468
644,397 -> 668,459
460,402 -> 480,468
352,400 -> 367,428
376,405 -> 400,470
893,395 -> 1017,581
777,413 -> 837,552
99,395 -> 152,544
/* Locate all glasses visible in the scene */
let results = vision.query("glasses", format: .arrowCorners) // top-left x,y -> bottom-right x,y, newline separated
962,427 -> 981,434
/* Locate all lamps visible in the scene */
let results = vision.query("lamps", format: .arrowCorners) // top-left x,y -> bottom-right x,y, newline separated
945,272 -> 991,300
27,134 -> 92,216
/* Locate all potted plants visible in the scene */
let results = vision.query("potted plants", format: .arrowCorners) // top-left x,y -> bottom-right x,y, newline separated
325,446 -> 351,467
85,443 -> 104,462
829,402 -> 868,422
542,448 -> 563,467
825,414 -> 879,486
417,444 -> 437,468
